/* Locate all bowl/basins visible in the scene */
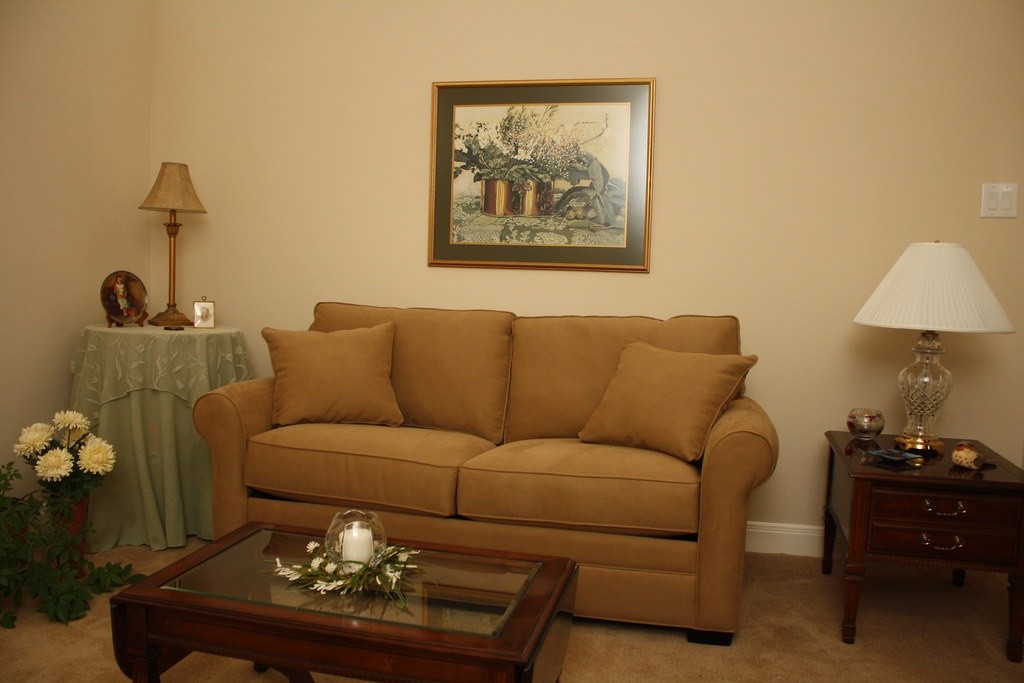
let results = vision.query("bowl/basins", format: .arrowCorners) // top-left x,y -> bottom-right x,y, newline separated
848,408 -> 885,441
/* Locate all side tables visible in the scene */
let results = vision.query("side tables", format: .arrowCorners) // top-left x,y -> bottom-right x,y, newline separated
65,323 -> 255,551
818,428 -> 1024,664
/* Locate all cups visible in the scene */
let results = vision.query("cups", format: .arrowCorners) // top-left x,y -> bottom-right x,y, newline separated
951,442 -> 985,470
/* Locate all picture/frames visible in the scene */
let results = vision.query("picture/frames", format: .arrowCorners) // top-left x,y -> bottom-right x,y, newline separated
99,270 -> 149,329
427,77 -> 656,274
192,295 -> 217,330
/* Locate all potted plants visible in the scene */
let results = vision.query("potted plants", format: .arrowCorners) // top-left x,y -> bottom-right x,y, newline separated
0,462 -> 149,630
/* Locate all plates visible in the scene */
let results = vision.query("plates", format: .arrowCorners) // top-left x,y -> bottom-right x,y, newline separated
867,448 -> 922,464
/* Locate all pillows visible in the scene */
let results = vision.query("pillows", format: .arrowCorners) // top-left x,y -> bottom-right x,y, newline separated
260,320 -> 404,427
578,337 -> 758,464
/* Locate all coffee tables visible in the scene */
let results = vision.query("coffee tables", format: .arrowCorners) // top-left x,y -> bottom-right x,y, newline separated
109,522 -> 579,683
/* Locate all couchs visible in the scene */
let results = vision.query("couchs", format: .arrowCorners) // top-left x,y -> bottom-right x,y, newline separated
192,300 -> 781,649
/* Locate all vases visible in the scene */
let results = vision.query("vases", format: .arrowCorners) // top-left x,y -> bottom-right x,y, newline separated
48,497 -> 90,580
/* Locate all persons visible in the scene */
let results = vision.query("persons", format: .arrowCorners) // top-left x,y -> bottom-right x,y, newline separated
114,275 -> 129,316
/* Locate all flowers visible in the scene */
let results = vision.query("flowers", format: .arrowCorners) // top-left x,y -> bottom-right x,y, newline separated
12,409 -> 118,503
255,539 -> 448,613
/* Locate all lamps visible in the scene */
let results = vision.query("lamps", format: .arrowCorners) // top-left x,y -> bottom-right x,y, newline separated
138,162 -> 208,325
850,239 -> 1015,454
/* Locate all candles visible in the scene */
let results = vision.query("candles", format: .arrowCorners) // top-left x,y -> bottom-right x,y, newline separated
323,509 -> 388,576
